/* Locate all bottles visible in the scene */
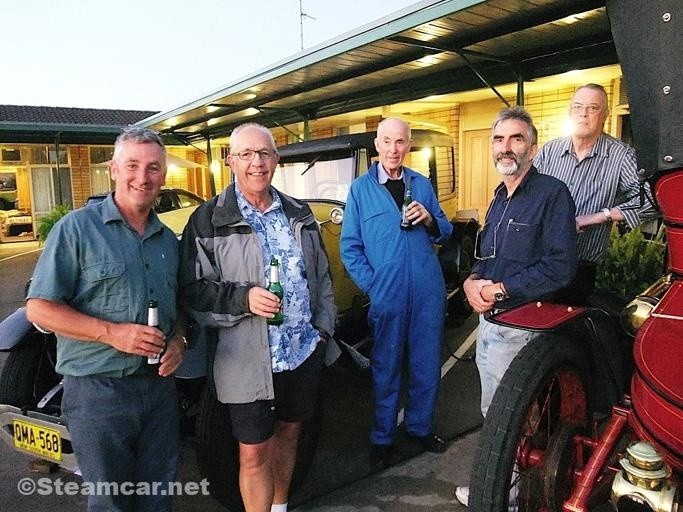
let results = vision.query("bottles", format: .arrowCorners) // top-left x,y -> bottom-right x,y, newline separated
398,191 -> 416,232
143,299 -> 167,369
265,260 -> 286,327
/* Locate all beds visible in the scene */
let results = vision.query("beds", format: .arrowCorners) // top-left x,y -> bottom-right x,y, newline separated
0,208 -> 32,237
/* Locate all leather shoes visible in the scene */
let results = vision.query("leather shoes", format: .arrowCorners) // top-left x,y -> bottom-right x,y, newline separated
409,429 -> 449,455
368,437 -> 405,466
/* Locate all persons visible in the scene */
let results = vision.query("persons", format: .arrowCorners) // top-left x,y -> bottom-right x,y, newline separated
337,115 -> 456,463
449,102 -> 583,511
23,125 -> 207,511
173,121 -> 338,511
526,83 -> 661,306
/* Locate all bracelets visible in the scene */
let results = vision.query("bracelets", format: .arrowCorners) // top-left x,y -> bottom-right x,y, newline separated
177,333 -> 189,350
600,207 -> 612,224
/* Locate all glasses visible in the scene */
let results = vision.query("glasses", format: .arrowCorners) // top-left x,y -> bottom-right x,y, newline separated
230,147 -> 275,161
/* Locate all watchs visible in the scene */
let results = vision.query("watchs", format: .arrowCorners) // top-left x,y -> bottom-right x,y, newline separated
493,282 -> 507,304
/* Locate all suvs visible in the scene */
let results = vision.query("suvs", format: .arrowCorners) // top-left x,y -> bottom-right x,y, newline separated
466,1 -> 683,511
82,186 -> 204,243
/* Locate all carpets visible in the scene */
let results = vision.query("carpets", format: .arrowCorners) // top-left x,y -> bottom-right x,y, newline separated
289,316 -> 485,511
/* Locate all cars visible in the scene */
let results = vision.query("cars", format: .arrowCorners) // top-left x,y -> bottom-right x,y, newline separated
0,197 -> 473,510
0,181 -> 17,202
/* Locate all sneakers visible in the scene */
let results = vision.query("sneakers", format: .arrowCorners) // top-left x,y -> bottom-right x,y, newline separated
453,484 -> 471,508
